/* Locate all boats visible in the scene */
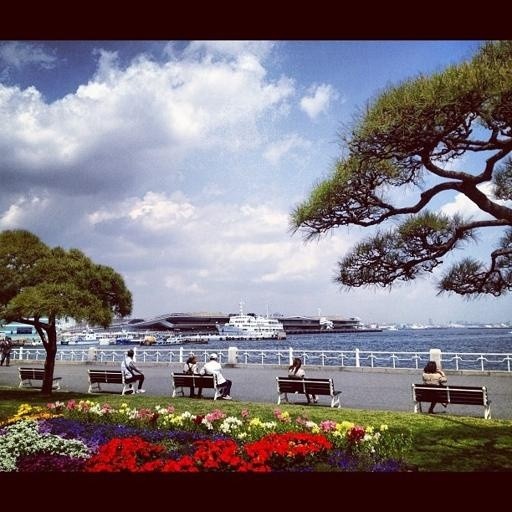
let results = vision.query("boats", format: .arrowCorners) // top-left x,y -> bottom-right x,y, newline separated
62,329 -> 210,345
215,295 -> 287,340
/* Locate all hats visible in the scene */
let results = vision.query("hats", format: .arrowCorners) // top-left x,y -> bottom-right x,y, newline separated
211,353 -> 218,359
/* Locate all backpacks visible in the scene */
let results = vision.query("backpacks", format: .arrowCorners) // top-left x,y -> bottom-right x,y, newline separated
186,364 -> 194,374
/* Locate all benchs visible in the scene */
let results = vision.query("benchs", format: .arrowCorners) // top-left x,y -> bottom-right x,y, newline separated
275,376 -> 342,410
88,368 -> 135,396
171,371 -> 226,400
412,383 -> 492,421
16,366 -> 62,392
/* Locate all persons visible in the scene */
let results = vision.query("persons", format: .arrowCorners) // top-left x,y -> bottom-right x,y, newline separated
422,361 -> 447,413
183,355 -> 204,398
200,353 -> 232,399
289,358 -> 319,403
0,336 -> 12,366
122,350 -> 146,393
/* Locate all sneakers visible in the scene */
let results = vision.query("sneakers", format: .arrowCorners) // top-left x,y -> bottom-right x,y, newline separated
128,387 -> 146,395
218,394 -> 231,400
